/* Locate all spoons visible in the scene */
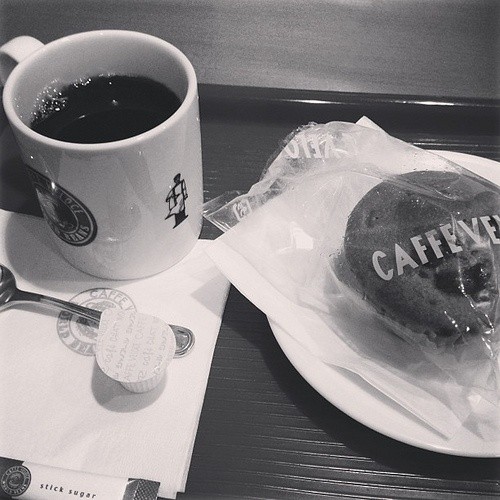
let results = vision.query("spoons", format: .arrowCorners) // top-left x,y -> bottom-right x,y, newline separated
0,265 -> 195,358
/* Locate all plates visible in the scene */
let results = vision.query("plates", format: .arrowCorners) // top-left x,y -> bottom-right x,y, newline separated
267,149 -> 500,458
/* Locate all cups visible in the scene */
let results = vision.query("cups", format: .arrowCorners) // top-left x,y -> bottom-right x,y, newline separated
0,30 -> 203,275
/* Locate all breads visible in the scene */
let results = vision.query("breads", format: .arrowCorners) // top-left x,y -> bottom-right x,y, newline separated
343,170 -> 500,350
